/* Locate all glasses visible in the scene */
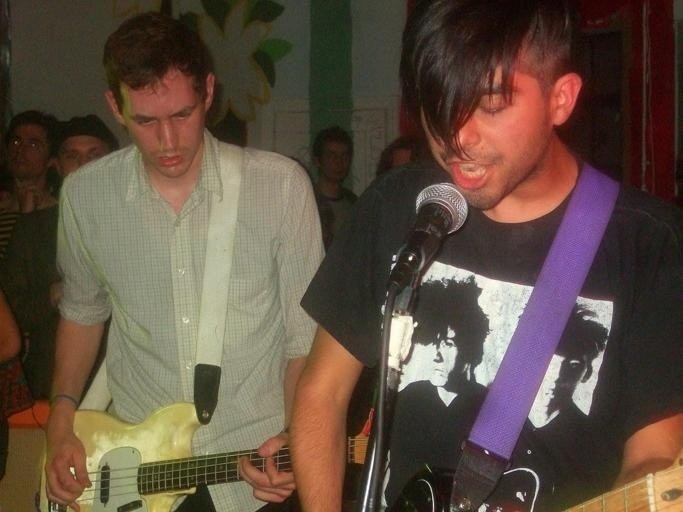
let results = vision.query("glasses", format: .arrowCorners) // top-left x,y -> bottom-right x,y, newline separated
9,138 -> 49,152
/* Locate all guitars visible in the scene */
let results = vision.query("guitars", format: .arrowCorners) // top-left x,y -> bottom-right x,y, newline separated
390,456 -> 683,512
38,403 -> 376,512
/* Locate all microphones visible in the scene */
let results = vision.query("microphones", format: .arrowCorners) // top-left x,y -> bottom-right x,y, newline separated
391,182 -> 469,289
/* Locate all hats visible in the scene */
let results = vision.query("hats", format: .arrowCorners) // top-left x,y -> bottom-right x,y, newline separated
53,115 -> 119,157
